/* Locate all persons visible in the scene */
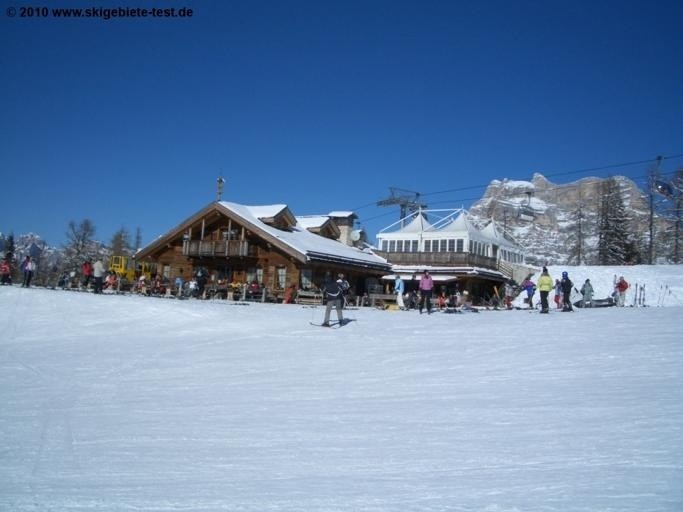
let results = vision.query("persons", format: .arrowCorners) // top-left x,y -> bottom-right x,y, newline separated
580,279 -> 595,308
418,269 -> 434,310
394,273 -> 408,311
283,285 -> 297,304
20,255 -> 34,287
615,276 -> 629,306
82,257 -> 92,289
0,256 -> 12,285
318,271 -> 346,326
92,257 -> 106,294
503,283 -> 514,307
522,277 -> 537,308
561,271 -> 574,312
537,266 -> 553,312
552,278 -> 563,309
335,271 -> 351,309
102,268 -> 264,299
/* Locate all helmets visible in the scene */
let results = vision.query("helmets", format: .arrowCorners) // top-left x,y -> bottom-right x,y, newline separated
563,272 -> 567,277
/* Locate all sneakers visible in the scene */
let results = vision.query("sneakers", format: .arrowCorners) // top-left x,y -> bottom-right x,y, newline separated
540,310 -> 548,313
322,323 -> 329,326
340,320 -> 345,325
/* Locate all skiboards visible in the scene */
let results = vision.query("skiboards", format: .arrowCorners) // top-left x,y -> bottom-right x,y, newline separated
310,318 -> 355,328
493,286 -> 504,307
635,283 -> 645,306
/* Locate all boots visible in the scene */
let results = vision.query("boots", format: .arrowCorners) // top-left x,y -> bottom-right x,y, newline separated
94,288 -> 102,293
562,303 -> 573,312
